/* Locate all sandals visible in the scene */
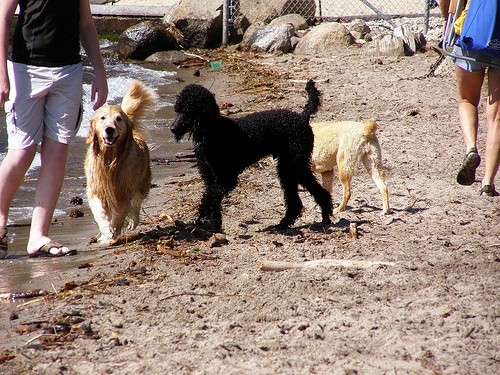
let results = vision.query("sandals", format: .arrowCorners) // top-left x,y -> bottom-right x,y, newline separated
481,184 -> 500,197
456,147 -> 481,187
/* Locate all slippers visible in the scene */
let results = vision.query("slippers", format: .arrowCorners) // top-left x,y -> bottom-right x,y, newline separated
0,228 -> 9,251
28,240 -> 78,258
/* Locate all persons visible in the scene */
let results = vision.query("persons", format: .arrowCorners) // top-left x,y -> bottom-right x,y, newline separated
0,0 -> 108,258
441,0 -> 500,196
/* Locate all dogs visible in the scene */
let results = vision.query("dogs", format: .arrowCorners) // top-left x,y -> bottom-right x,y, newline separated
81,78 -> 161,248
307,118 -> 393,218
168,76 -> 336,240
